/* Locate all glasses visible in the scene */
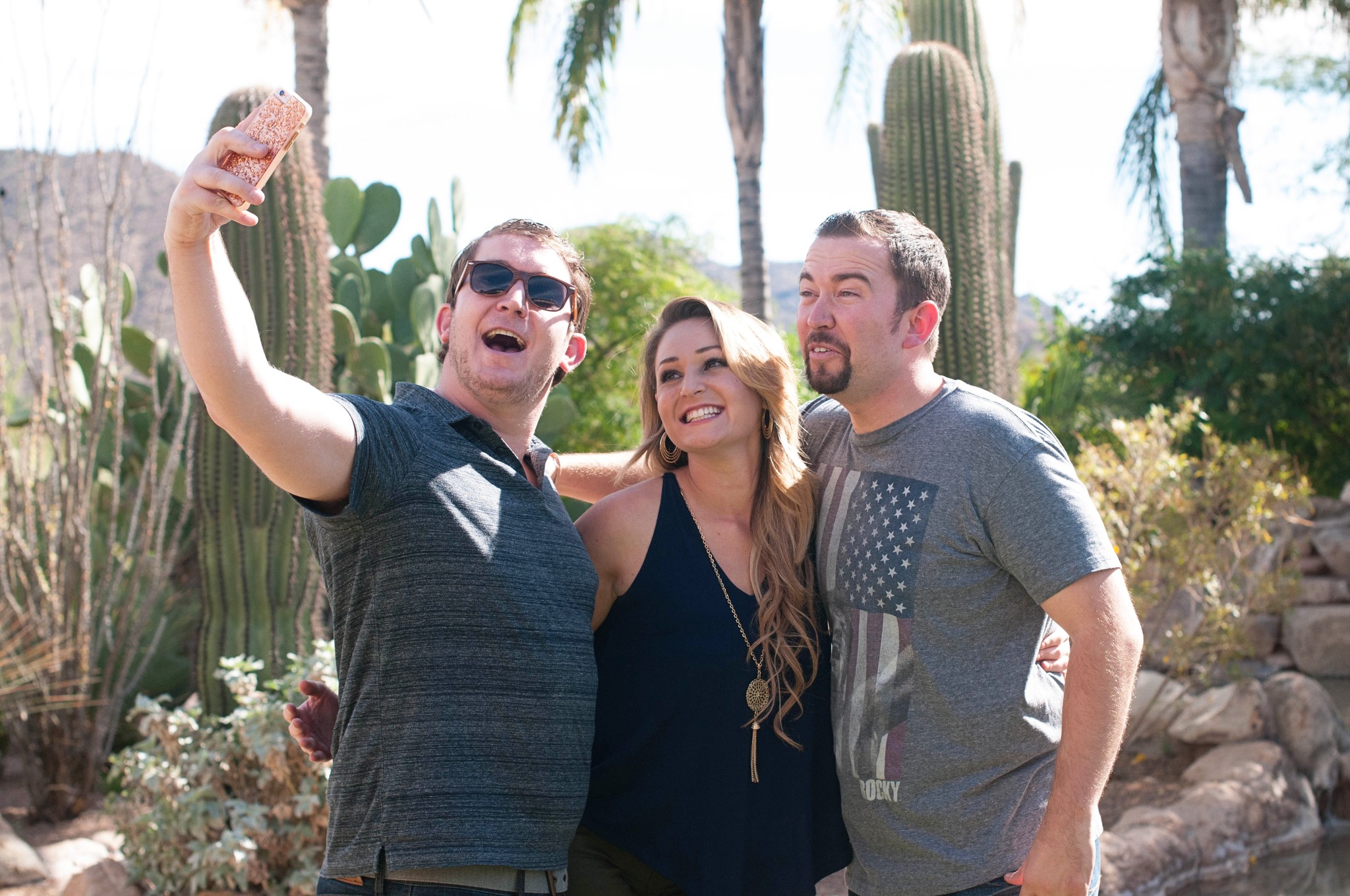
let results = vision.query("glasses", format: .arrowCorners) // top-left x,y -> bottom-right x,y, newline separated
454,260 -> 578,323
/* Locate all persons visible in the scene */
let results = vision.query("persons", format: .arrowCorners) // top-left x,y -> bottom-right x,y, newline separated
164,112 -> 1145,896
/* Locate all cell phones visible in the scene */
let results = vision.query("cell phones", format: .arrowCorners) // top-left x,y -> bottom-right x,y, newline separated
219,86 -> 312,213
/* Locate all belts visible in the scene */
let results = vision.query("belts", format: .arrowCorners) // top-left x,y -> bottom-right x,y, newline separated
364,863 -> 568,895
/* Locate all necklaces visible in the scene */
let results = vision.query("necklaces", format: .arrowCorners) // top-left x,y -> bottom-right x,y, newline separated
677,481 -> 776,785
